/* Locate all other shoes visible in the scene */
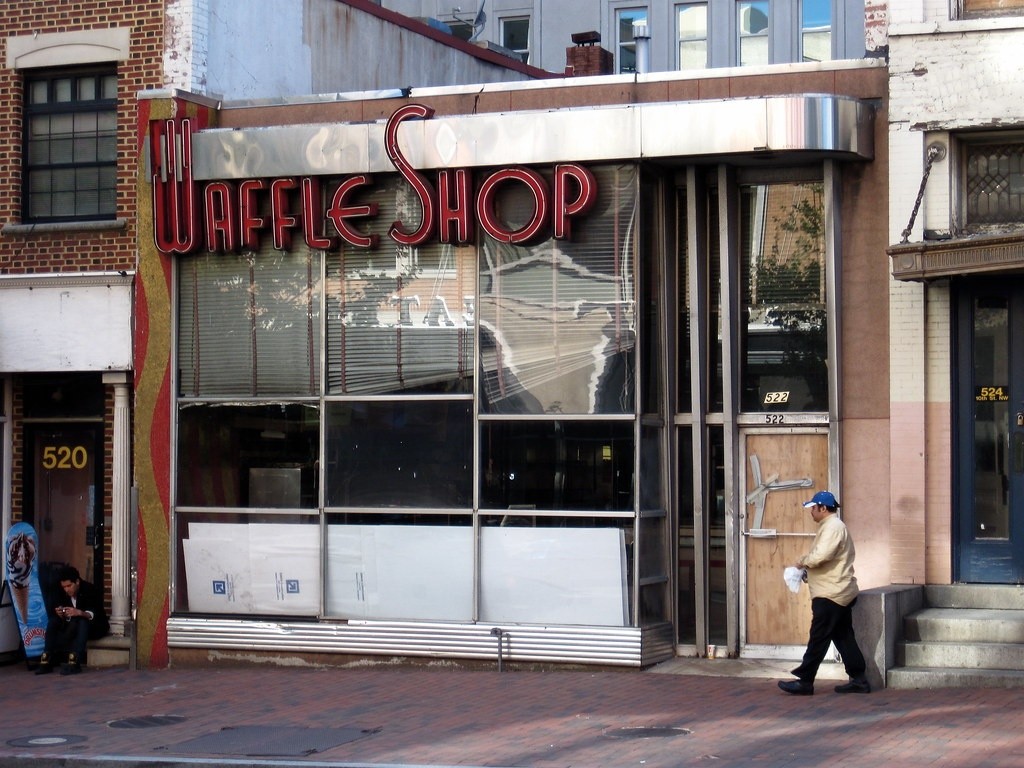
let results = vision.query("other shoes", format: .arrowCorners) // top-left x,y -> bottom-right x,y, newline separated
60,663 -> 81,674
36,665 -> 53,674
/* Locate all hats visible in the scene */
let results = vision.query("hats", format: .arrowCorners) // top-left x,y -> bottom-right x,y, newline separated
802,491 -> 840,508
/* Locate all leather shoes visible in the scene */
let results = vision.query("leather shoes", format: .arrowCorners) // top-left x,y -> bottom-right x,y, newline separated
834,682 -> 870,692
778,680 -> 815,695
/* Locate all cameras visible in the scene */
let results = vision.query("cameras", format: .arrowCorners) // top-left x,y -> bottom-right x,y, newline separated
59,608 -> 67,613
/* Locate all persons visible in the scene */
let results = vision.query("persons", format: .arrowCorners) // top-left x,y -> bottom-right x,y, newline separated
35,567 -> 113,675
778,491 -> 871,695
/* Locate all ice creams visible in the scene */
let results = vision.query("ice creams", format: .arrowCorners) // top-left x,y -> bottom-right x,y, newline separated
8,531 -> 36,625
